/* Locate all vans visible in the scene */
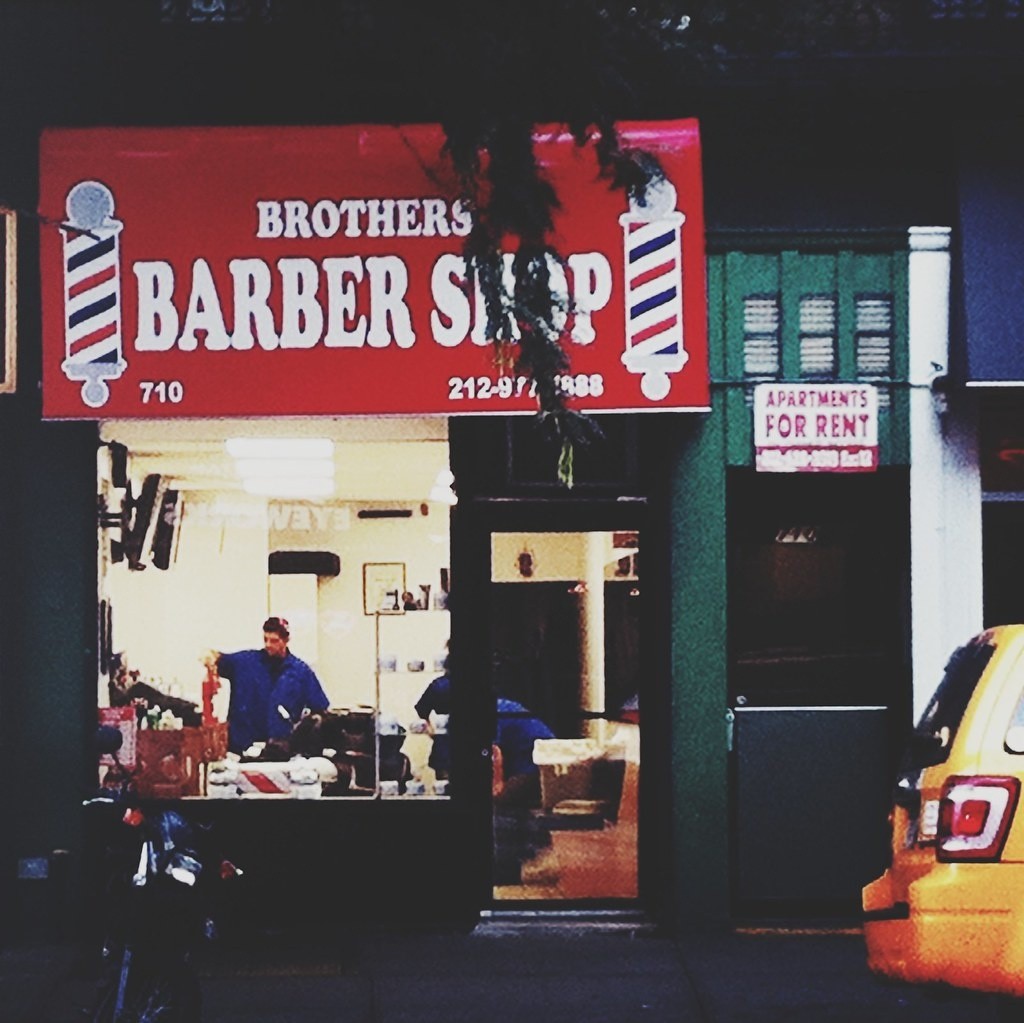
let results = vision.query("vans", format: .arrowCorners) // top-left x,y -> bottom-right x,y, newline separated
861,620 -> 1023,999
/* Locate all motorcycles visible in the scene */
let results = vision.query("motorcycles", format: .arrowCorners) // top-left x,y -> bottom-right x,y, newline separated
0,727 -> 260,1023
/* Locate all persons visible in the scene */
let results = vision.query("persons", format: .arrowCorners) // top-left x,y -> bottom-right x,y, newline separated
414,637 -> 453,779
204,617 -> 330,757
493,696 -> 557,810
